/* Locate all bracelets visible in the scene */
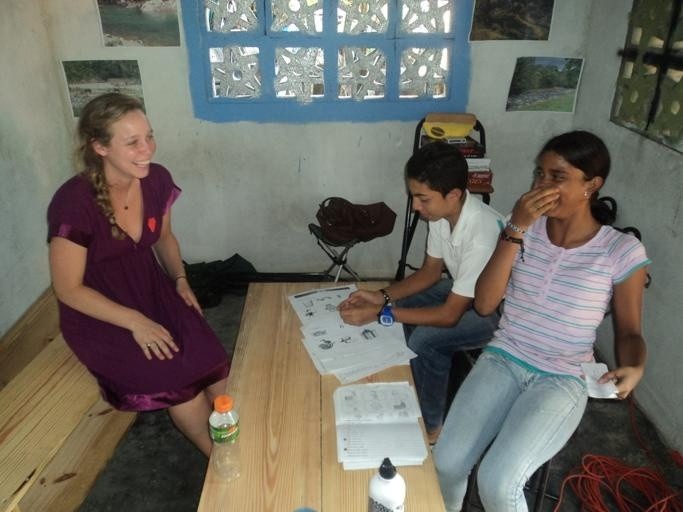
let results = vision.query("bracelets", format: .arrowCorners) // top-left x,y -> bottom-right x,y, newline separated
508,220 -> 525,235
173,274 -> 187,283
500,230 -> 524,245
376,305 -> 384,323
376,289 -> 392,305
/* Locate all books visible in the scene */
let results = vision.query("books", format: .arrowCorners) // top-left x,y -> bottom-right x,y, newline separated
419,134 -> 492,190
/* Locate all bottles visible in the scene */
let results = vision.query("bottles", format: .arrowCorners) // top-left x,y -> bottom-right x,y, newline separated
367,458 -> 406,512
208,395 -> 241,482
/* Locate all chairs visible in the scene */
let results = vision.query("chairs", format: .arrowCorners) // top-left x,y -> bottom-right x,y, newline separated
396,114 -> 496,283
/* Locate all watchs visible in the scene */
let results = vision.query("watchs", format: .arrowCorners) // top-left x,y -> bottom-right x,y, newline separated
380,303 -> 395,327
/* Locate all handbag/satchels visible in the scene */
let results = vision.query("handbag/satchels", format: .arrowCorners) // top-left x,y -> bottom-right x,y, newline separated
316,197 -> 398,242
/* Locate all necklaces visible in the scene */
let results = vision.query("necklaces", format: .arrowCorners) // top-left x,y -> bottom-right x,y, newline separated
104,184 -> 132,212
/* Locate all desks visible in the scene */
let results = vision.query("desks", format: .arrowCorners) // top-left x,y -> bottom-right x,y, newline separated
198,280 -> 446,512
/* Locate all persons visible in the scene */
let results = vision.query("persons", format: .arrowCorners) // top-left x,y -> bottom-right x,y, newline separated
43,92 -> 231,459
334,141 -> 509,453
430,130 -> 654,512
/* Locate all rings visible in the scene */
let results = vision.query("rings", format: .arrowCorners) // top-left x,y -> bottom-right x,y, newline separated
146,340 -> 156,347
